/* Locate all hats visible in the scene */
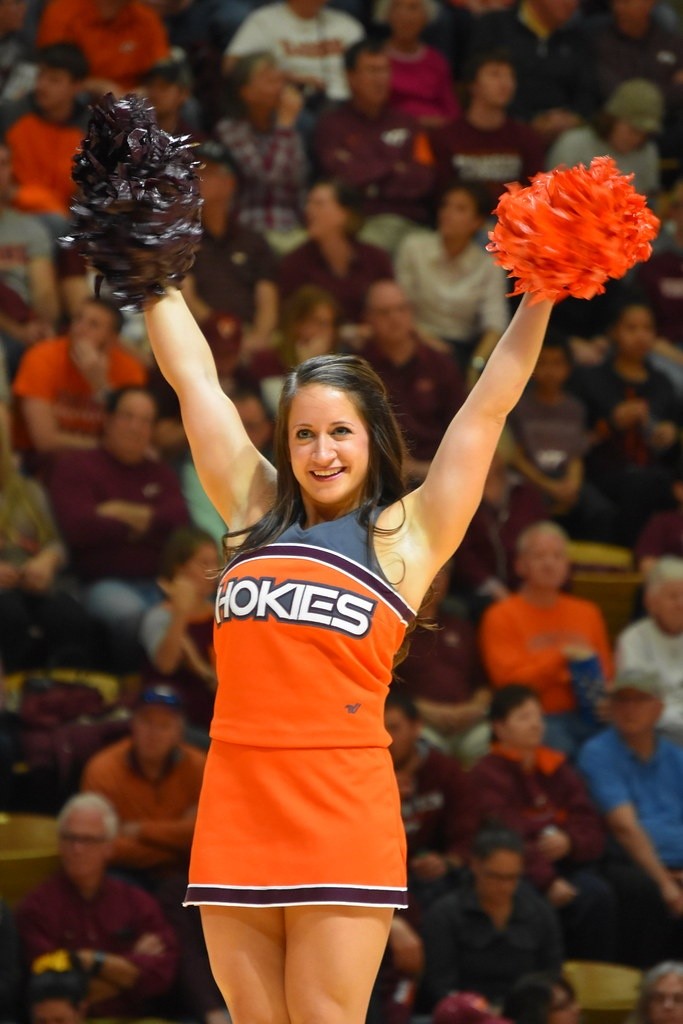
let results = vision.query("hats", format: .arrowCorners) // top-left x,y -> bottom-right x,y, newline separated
603,668 -> 663,699
604,78 -> 665,136
132,682 -> 185,706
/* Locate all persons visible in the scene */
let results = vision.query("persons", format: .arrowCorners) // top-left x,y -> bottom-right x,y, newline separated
52,87 -> 659,1023
0,0 -> 683,1024
